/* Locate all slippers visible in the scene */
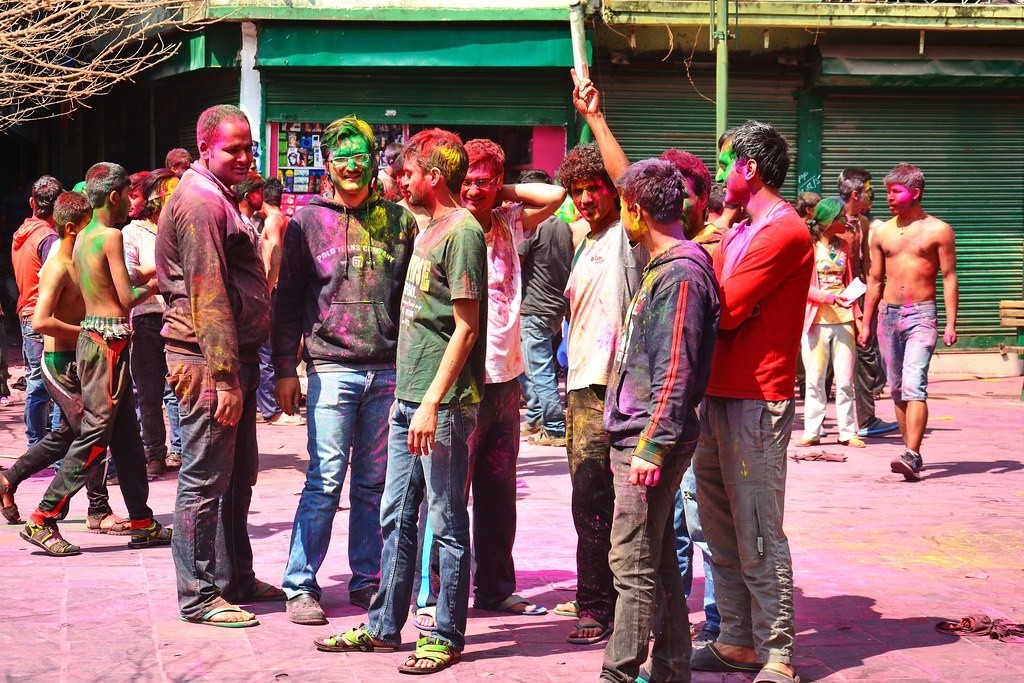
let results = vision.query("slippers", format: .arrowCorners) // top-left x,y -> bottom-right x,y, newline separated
226,584 -> 288,604
398,631 -> 461,674
473,594 -> 547,615
314,623 -> 395,653
285,593 -> 325,624
566,623 -> 612,644
270,410 -> 307,426
751,665 -> 801,683
693,623 -> 721,646
628,666 -> 658,683
414,605 -> 439,631
553,599 -> 615,622
688,642 -> 764,672
350,586 -> 381,611
795,418 -> 899,448
180,604 -> 259,628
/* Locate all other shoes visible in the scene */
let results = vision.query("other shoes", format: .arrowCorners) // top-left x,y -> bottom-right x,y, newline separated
0,396 -> 14,407
103,450 -> 182,487
520,416 -> 567,446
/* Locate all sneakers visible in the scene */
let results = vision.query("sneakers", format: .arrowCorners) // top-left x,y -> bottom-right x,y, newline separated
890,449 -> 923,480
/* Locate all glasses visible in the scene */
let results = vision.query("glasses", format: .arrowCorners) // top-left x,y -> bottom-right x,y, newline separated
326,153 -> 371,169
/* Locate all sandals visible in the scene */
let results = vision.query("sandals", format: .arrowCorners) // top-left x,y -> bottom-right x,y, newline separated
127,519 -> 173,548
0,466 -> 20,524
18,517 -> 80,556
85,510 -> 134,535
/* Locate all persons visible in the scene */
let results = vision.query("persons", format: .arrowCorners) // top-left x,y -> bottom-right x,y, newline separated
691,125 -> 814,682
156,106 -> 287,626
459,139 -> 567,616
314,128 -> 489,675
595,158 -> 718,682
800,196 -> 867,448
269,116 -> 418,625
833,168 -> 899,436
658,147 -> 723,646
706,183 -> 737,232
792,191 -> 821,223
856,163 -> 958,479
2,148 -> 434,555
522,166 -> 576,445
560,63 -> 643,642
